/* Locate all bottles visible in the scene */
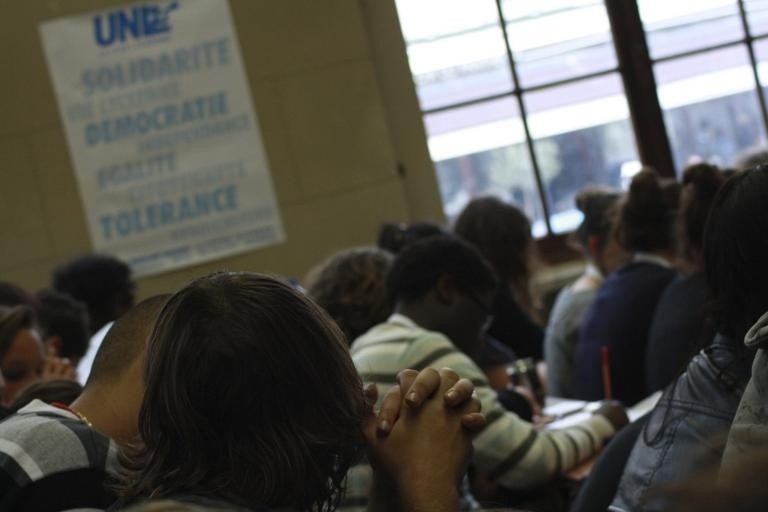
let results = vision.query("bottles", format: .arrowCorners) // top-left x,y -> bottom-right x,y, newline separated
503,355 -> 546,410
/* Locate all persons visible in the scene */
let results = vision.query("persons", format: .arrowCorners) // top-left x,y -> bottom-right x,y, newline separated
0,146 -> 768,511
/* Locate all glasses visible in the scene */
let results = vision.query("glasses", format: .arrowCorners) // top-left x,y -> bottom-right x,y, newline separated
458,283 -> 495,331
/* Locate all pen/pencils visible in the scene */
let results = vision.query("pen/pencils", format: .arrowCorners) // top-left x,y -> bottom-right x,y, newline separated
596,345 -> 620,404
